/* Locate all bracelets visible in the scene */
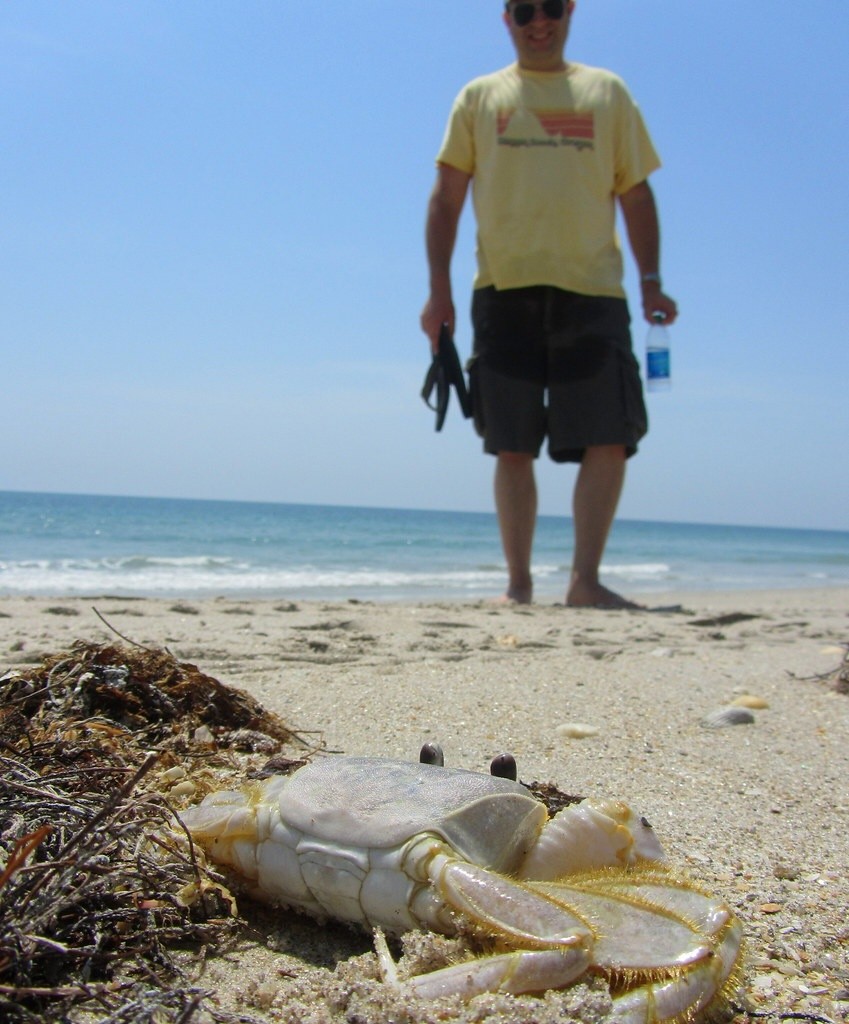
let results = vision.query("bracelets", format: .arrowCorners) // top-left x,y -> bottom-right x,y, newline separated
642,273 -> 659,282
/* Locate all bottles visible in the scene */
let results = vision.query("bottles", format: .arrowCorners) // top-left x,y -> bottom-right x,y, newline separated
645,310 -> 671,393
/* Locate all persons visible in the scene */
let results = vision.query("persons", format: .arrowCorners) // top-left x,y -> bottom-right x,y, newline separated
419,0 -> 680,610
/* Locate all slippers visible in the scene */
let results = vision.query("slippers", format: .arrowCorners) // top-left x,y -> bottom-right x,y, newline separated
421,320 -> 471,432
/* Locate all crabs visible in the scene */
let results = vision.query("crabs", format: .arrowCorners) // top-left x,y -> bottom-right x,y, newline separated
151,740 -> 748,1024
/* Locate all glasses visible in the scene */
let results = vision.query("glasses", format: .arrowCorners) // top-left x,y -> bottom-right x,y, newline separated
513,0 -> 570,26
